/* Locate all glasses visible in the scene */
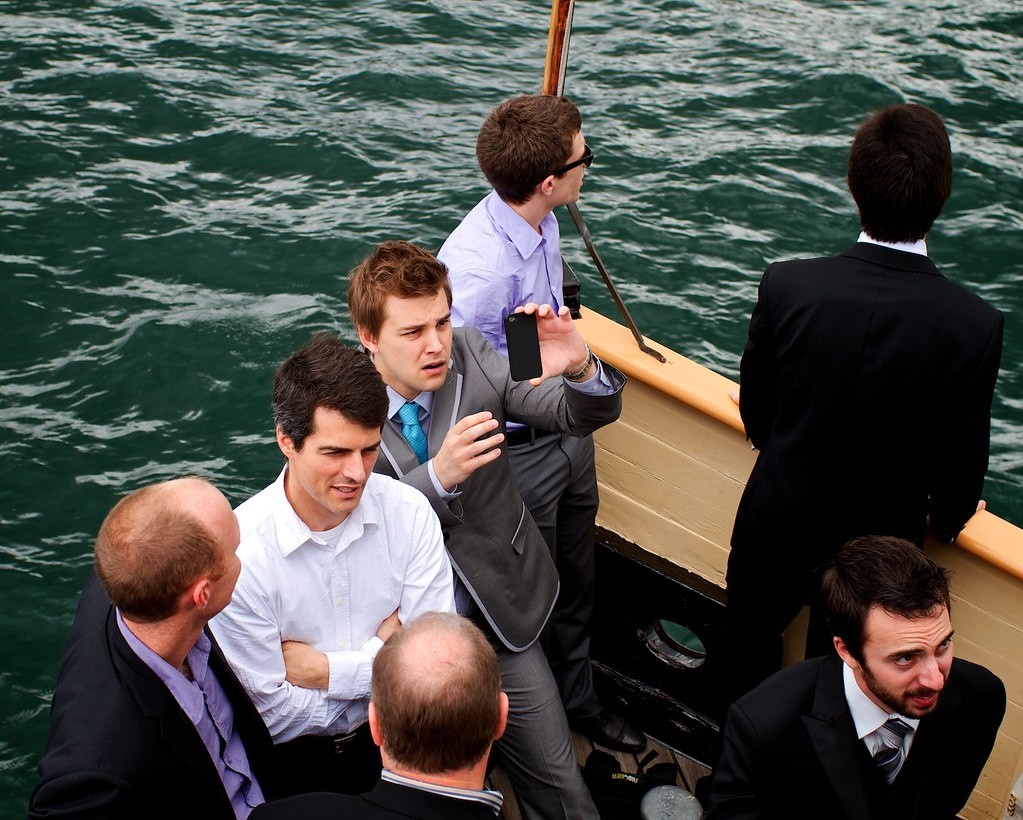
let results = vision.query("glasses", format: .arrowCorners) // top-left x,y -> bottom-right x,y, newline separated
550,145 -> 594,178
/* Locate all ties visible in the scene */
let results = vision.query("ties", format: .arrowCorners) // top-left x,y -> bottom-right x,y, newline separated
873,718 -> 912,787
398,403 -> 428,465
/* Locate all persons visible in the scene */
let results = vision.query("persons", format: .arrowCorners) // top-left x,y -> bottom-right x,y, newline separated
711,104 -> 1005,731
699,534 -> 1005,819
434,96 -> 645,757
346,243 -> 628,820
29,477 -> 286,820
247,611 -> 511,820
208,333 -> 456,793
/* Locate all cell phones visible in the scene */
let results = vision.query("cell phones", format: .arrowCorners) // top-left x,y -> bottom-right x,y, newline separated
504,311 -> 544,384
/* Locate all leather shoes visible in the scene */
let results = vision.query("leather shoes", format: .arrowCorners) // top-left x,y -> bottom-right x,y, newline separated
567,708 -> 647,755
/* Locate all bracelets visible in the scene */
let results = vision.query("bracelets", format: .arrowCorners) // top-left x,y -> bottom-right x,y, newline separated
563,344 -> 594,380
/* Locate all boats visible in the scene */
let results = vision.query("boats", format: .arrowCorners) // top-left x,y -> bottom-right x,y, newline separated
531,0 -> 1023,818
